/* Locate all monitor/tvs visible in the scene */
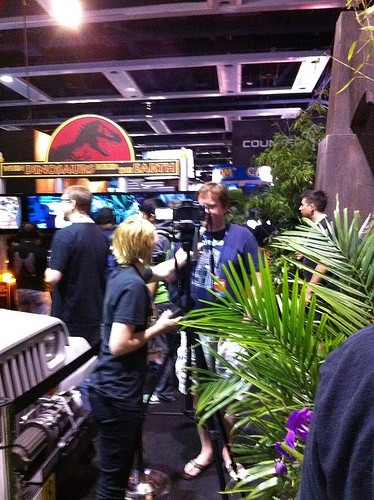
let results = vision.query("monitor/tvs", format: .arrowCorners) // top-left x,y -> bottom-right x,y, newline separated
0,191 -> 199,233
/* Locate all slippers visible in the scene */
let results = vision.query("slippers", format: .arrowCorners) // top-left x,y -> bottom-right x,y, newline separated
182,459 -> 205,479
222,463 -> 245,480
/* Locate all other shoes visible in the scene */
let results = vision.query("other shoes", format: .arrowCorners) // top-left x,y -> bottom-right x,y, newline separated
142,394 -> 160,404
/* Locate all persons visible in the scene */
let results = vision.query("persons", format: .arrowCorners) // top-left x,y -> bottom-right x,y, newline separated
163,182 -> 265,481
94,196 -> 200,403
86,215 -> 187,500
5,222 -> 48,315
44,185 -> 111,466
298,190 -> 340,340
240,207 -> 277,248
296,325 -> 374,500
